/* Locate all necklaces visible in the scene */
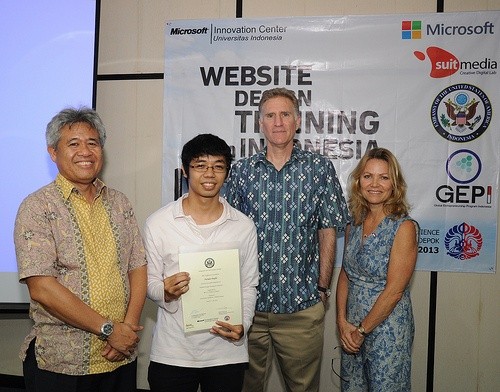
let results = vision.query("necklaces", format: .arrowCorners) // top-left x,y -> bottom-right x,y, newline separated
364,233 -> 368,237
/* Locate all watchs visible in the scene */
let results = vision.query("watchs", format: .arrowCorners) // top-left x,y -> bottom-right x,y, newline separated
97,318 -> 114,341
358,323 -> 369,337
318,286 -> 331,297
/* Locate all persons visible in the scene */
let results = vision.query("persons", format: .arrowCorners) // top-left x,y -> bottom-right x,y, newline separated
146,134 -> 260,392
14,106 -> 148,392
226,88 -> 350,392
336,148 -> 420,392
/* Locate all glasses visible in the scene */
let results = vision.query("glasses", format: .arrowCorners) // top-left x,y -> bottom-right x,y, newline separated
188,163 -> 228,173
